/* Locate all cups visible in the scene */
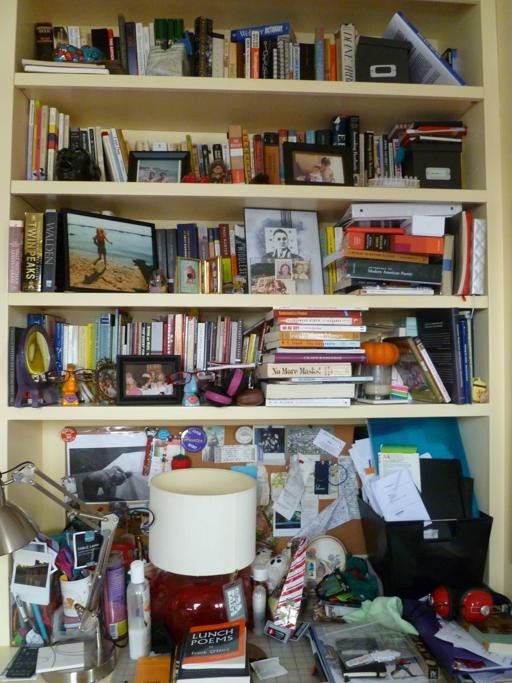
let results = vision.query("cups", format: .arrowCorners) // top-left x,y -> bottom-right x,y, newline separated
60,567 -> 92,617
362,364 -> 392,400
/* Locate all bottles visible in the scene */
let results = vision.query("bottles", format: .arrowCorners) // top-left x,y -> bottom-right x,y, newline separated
125,560 -> 153,660
100,551 -> 128,640
250,565 -> 269,633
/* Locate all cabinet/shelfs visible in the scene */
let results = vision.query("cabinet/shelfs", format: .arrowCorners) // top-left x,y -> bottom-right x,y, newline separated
0,0 -> 502,646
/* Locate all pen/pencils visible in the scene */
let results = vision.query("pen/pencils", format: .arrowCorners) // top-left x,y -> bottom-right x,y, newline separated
60,569 -> 80,582
312,653 -> 319,676
12,594 -> 50,645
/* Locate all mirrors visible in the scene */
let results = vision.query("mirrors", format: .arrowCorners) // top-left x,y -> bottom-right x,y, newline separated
14,324 -> 61,406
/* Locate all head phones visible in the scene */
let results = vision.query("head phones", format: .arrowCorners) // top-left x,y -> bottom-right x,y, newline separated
429,582 -> 511,622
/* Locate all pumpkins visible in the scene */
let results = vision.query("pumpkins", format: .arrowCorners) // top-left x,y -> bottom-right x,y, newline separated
361,342 -> 399,365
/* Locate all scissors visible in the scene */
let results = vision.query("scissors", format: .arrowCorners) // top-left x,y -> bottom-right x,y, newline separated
56,548 -> 74,581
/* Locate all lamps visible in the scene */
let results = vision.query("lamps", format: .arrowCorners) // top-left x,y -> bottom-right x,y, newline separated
1,460 -> 122,681
144,462 -> 261,653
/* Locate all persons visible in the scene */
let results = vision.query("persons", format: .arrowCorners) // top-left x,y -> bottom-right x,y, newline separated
144,168 -> 168,183
272,230 -> 308,279
130,371 -> 172,395
93,227 -> 113,270
309,157 -> 335,183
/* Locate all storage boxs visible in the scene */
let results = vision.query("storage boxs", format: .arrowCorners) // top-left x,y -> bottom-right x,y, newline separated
354,489 -> 496,610
355,35 -> 413,82
403,142 -> 463,189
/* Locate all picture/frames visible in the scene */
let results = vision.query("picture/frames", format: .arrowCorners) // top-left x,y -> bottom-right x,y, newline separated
59,207 -> 159,292
282,141 -> 354,186
203,253 -> 224,296
174,253 -> 203,295
114,352 -> 184,406
242,204 -> 327,295
128,149 -> 191,182
91,357 -> 117,404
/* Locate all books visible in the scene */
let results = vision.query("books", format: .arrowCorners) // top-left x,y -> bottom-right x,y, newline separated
377,443 -> 422,494
9,209 -> 59,293
457,611 -> 512,654
21,12 -> 356,82
168,618 -> 251,677
135,141 -> 188,151
121,313 -> 265,373
35,640 -> 86,674
317,115 -> 347,147
372,308 -> 474,405
9,308 -> 132,407
26,100 -> 129,182
381,12 -> 465,86
156,222 -> 248,294
318,202 -> 485,294
348,115 -> 467,188
309,623 -> 431,683
186,125 -> 315,185
254,306 -> 374,406
